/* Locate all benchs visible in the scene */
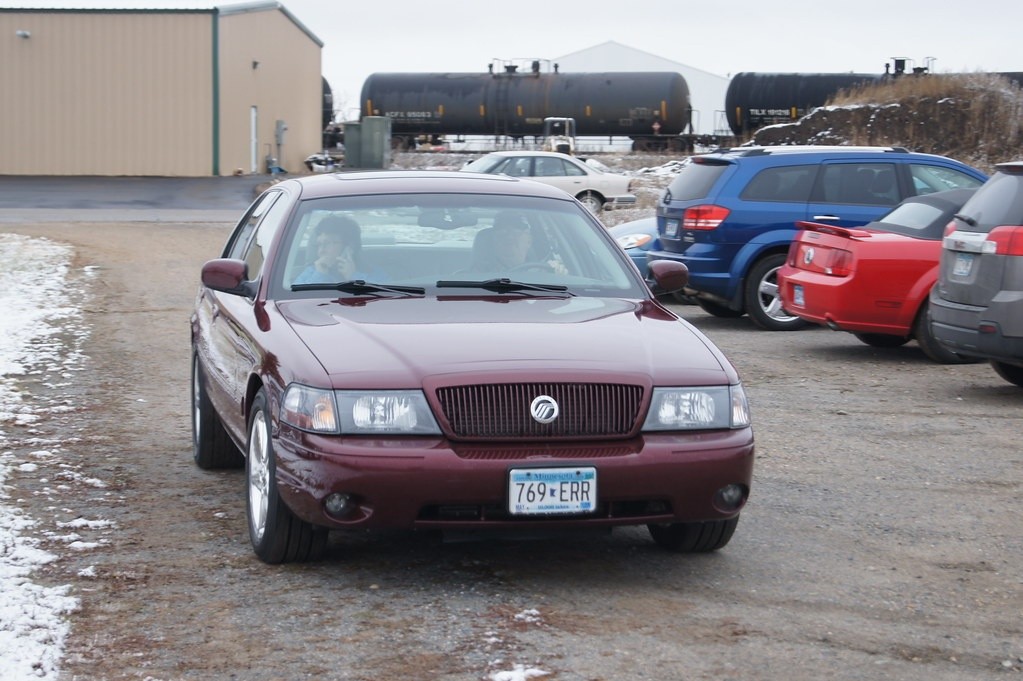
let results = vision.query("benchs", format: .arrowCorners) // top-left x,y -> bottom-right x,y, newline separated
296,244 -> 475,278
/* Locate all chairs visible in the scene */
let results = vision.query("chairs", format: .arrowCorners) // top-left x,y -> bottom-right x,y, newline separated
471,227 -> 495,266
306,223 -> 367,271
850,169 -> 894,206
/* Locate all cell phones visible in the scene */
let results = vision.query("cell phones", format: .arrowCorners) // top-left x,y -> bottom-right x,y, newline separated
341,247 -> 354,262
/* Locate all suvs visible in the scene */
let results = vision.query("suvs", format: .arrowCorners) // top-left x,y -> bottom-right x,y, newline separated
645,143 -> 995,333
925,160 -> 1023,387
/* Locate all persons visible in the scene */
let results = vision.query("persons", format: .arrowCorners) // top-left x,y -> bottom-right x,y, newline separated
449,212 -> 569,282
290,213 -> 383,287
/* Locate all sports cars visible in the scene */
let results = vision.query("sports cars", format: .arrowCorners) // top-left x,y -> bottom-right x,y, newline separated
775,185 -> 984,365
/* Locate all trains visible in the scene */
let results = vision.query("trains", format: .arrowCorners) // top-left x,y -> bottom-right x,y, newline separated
321,59 -> 1023,155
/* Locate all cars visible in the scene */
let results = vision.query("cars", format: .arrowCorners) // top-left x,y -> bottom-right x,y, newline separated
459,150 -> 637,217
603,215 -> 661,281
187,169 -> 758,563
